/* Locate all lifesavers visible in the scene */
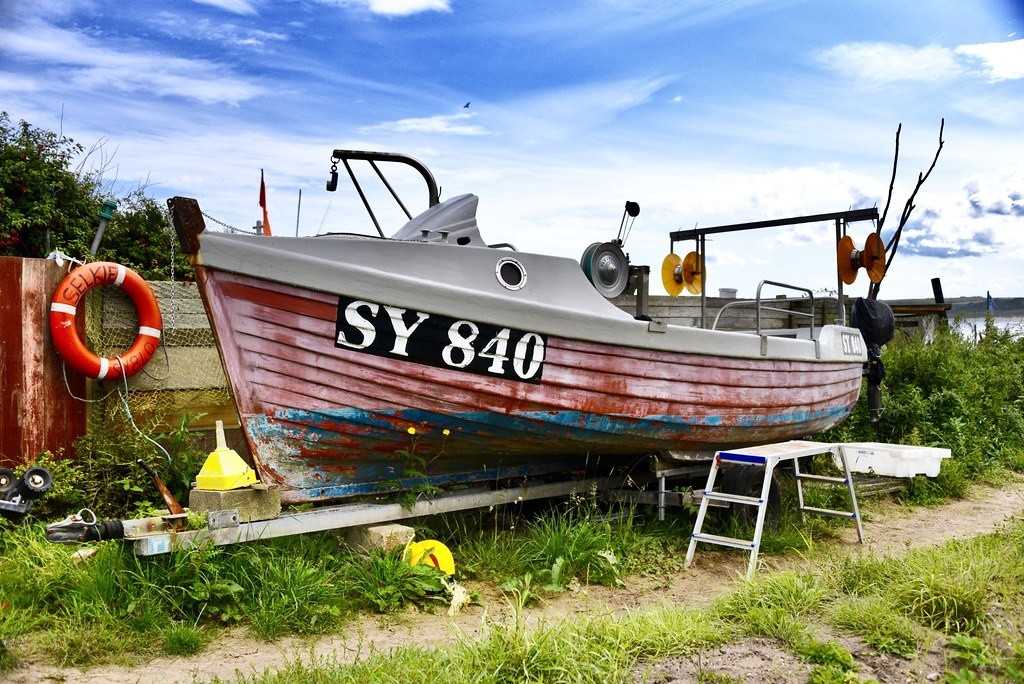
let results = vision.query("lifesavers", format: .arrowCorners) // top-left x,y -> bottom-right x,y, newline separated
50,261 -> 164,381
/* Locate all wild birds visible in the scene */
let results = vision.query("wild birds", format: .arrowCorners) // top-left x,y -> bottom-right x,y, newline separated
464,102 -> 470,108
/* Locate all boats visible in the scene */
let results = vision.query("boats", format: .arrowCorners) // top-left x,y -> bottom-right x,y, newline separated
167,149 -> 878,506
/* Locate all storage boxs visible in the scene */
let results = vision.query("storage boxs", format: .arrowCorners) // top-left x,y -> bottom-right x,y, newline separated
832,442 -> 952,478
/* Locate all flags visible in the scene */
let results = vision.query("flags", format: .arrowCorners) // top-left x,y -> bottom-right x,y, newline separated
260,178 -> 271,236
